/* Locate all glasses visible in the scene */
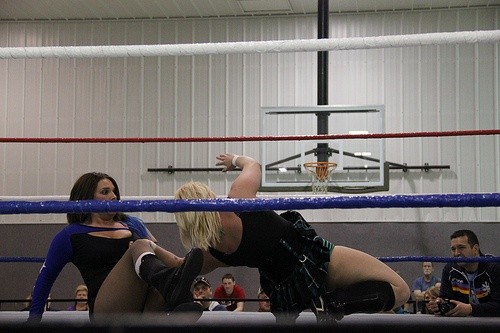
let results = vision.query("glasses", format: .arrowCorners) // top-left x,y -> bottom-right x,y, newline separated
261,296 -> 270,299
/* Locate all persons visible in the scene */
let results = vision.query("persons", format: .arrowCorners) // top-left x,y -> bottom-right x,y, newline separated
128,151 -> 411,329
426,229 -> 500,317
27,172 -> 205,328
22,259 -> 443,314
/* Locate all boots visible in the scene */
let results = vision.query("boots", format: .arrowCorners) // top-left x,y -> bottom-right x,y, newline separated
135,248 -> 204,306
168,291 -> 203,327
271,304 -> 308,326
310,281 -> 396,326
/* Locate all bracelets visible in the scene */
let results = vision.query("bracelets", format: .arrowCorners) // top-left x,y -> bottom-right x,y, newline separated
231,155 -> 240,167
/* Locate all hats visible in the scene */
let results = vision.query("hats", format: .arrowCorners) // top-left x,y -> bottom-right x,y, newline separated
193,276 -> 211,289
257,287 -> 263,298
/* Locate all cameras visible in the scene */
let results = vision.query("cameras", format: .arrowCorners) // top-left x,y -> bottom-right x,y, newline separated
437,302 -> 456,315
